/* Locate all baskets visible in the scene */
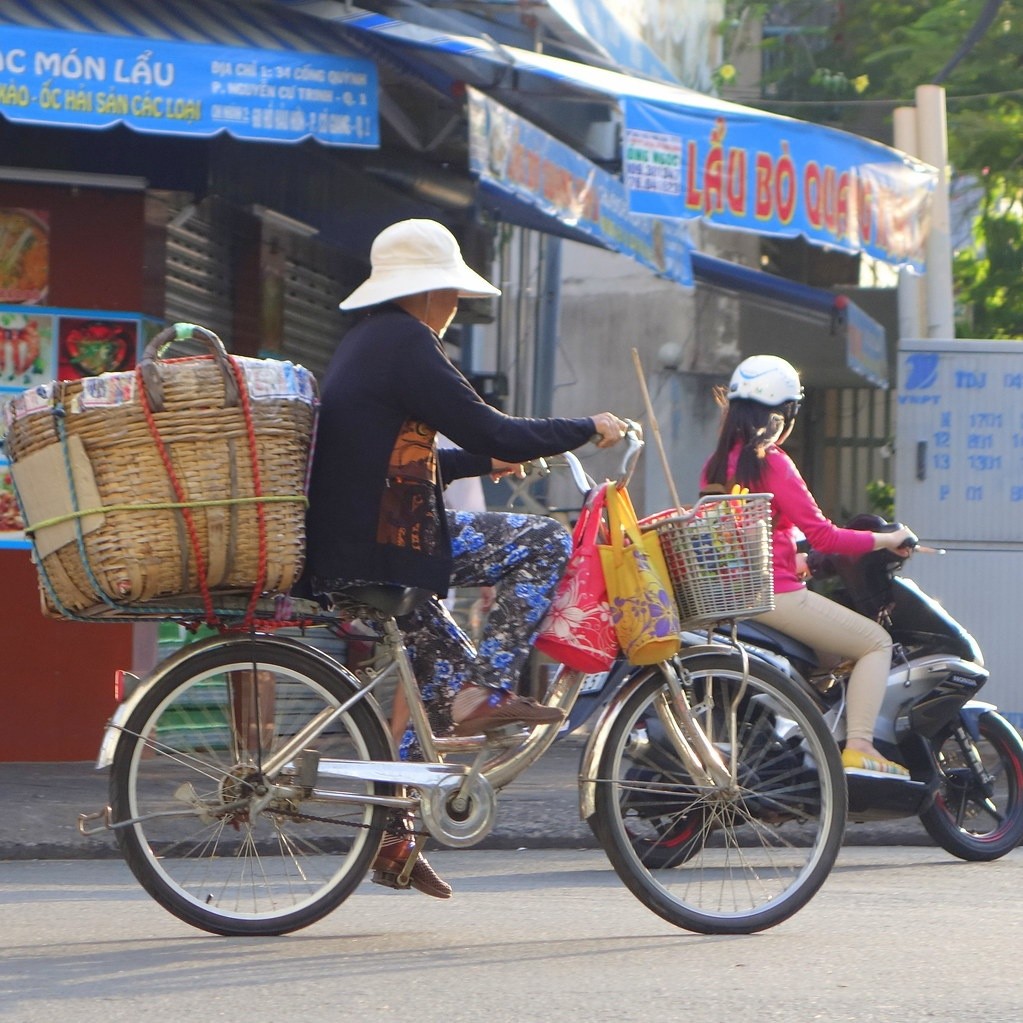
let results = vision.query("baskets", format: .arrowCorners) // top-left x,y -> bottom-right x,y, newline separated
4,323 -> 319,616
637,492 -> 776,631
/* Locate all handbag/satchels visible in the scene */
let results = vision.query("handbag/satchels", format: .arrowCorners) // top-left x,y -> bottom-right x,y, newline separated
534,483 -> 621,674
598,481 -> 681,665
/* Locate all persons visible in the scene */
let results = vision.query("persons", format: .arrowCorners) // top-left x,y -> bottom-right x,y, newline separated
298,217 -> 630,898
696,351 -> 921,786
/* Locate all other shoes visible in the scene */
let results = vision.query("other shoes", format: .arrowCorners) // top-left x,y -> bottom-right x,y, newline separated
842,747 -> 912,781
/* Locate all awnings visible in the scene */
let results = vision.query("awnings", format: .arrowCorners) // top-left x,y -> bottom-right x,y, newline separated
0,1 -> 944,390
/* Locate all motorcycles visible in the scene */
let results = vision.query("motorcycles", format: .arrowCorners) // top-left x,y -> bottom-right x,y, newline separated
553,513 -> 1023,870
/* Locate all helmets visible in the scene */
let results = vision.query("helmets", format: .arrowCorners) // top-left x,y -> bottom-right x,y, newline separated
727,355 -> 804,406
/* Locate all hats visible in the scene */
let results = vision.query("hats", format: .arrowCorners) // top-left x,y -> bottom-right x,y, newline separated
339,219 -> 502,311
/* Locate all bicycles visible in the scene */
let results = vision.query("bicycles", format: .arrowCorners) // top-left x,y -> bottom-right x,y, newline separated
79,417 -> 847,936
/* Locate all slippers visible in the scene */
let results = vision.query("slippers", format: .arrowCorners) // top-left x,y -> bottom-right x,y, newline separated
454,690 -> 566,736
373,852 -> 452,898
